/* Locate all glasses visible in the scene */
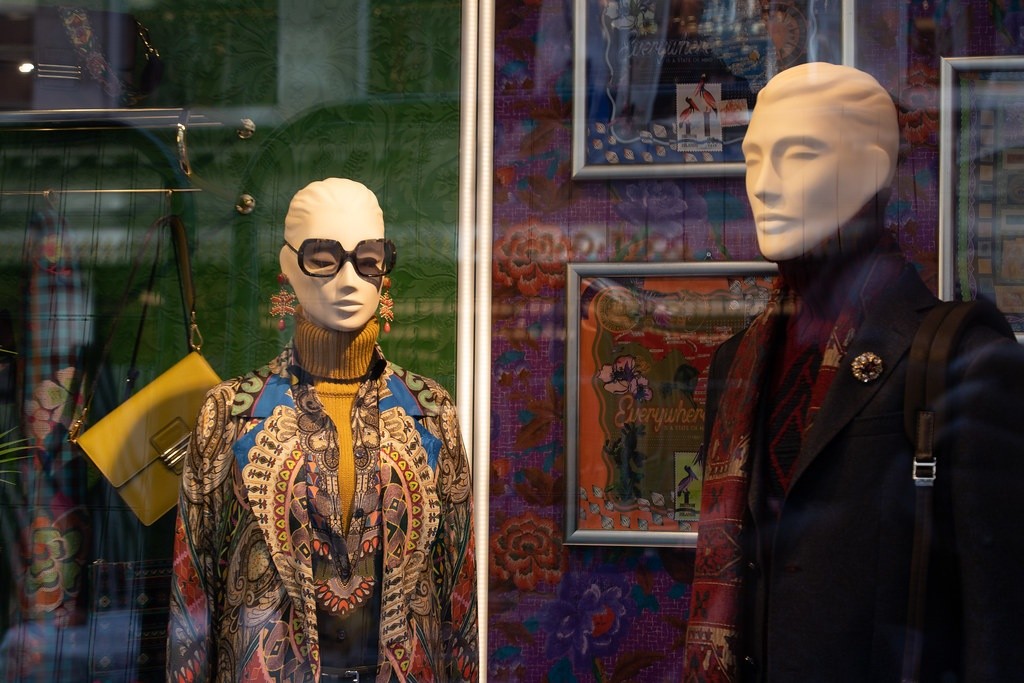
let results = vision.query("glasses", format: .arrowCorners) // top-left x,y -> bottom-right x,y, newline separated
283,238 -> 397,278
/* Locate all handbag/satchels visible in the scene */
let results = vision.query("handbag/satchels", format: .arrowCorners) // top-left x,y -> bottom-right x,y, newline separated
67,214 -> 224,527
26,4 -> 164,109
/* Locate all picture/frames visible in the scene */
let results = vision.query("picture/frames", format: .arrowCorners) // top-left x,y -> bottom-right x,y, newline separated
567,262 -> 778,547
938,55 -> 1023,347
571,0 -> 854,181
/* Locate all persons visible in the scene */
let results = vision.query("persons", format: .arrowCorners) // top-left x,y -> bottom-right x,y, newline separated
166,176 -> 480,683
700,62 -> 1024,683
